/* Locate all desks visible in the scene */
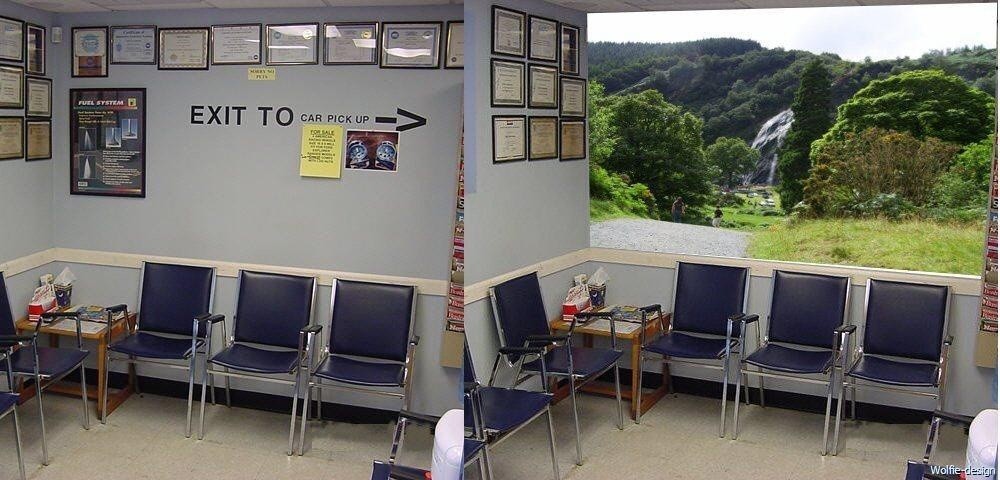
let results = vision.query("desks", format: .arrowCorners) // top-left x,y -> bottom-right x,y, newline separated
548,310 -> 672,420
15,305 -> 137,421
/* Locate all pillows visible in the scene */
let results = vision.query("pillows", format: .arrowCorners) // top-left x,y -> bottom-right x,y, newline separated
965,411 -> 997,479
429,410 -> 462,479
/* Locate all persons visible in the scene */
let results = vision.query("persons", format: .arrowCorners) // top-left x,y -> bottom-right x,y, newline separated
712,205 -> 723,233
671,195 -> 685,226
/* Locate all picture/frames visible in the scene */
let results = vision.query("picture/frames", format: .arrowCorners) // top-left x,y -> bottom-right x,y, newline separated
490,3 -> 588,164
69,88 -> 145,198
0,14 -> 53,160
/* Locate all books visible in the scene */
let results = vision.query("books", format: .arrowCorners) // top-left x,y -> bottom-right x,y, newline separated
70,296 -> 118,326
610,304 -> 656,325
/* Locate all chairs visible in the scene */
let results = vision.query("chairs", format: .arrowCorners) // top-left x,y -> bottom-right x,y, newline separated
830,277 -> 954,465
464,336 -> 559,480
905,410 -> 997,479
0,271 -> 91,468
636,260 -> 752,438
101,258 -> 219,437
731,269 -> 852,455
371,410 -> 464,480
298,278 -> 420,462
490,271 -> 626,467
463,386 -> 489,480
197,266 -> 319,455
0,339 -> 26,478
